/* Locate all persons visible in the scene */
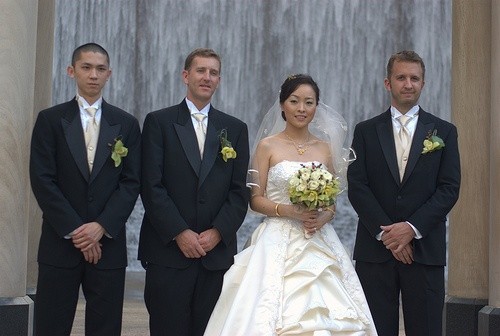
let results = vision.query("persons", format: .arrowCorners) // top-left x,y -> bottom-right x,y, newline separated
347,50 -> 462,336
137,49 -> 250,336
29,41 -> 141,336
250,74 -> 338,336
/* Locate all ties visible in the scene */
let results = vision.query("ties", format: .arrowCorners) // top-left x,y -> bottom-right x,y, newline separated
85,107 -> 98,142
192,113 -> 207,159
396,115 -> 411,152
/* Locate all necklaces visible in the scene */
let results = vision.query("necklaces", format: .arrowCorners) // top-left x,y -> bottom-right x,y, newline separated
284,127 -> 310,155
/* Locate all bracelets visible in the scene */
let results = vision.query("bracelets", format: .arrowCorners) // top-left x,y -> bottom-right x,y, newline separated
324,207 -> 336,214
275,203 -> 281,216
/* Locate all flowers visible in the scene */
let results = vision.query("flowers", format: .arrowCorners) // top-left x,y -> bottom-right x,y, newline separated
289,162 -> 340,239
220,137 -> 237,162
422,133 -> 448,154
111,139 -> 129,168
